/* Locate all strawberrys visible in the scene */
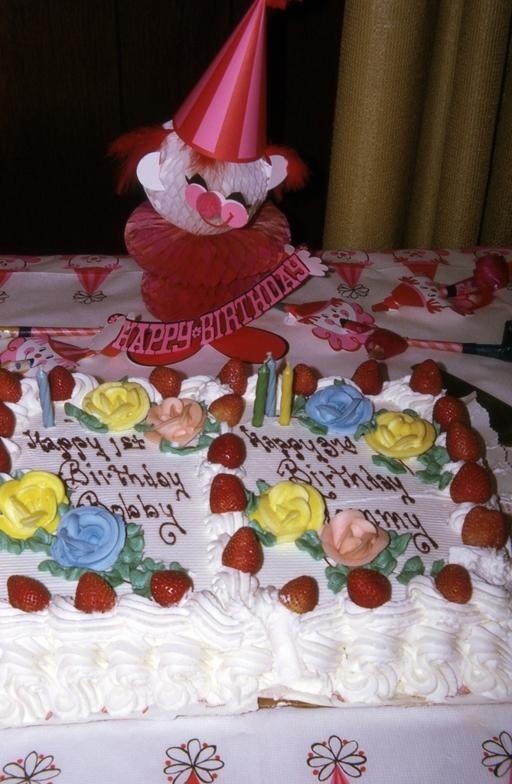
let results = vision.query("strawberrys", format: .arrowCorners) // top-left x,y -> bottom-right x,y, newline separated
365,328 -> 409,359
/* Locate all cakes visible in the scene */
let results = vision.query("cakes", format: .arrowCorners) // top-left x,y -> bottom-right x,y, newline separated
0,358 -> 512,727
